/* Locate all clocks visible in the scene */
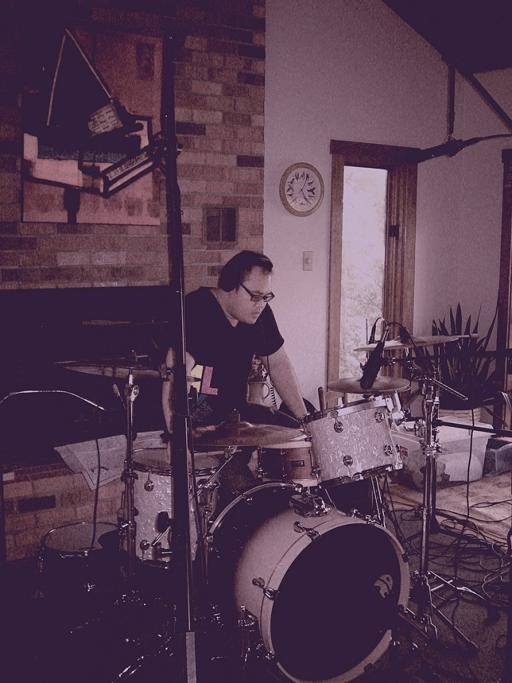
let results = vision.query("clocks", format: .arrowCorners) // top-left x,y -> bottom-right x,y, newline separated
278,162 -> 326,216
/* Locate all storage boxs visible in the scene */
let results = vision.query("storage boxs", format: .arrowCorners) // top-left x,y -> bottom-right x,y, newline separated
386,416 -> 512,489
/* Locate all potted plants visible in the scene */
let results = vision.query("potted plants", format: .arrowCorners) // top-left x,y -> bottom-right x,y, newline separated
413,303 -> 511,422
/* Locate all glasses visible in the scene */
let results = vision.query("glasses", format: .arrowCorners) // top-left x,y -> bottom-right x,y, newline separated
242,283 -> 275,302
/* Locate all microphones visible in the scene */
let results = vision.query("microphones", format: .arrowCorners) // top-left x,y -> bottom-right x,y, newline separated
359,327 -> 388,389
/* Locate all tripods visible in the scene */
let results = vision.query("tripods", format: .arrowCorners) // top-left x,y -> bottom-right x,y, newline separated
399,401 -> 485,653
61,387 -> 228,681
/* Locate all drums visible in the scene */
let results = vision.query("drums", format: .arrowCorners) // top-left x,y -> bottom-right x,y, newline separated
119,447 -> 222,570
41,521 -> 118,591
205,483 -> 410,683
257,427 -> 323,488
298,395 -> 404,489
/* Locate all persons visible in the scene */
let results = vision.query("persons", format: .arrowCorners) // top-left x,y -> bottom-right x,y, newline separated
162,250 -> 308,496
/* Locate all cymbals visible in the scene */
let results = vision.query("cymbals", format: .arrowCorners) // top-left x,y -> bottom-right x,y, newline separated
63,358 -> 199,383
188,421 -> 307,448
327,374 -> 411,394
353,334 -> 460,352
